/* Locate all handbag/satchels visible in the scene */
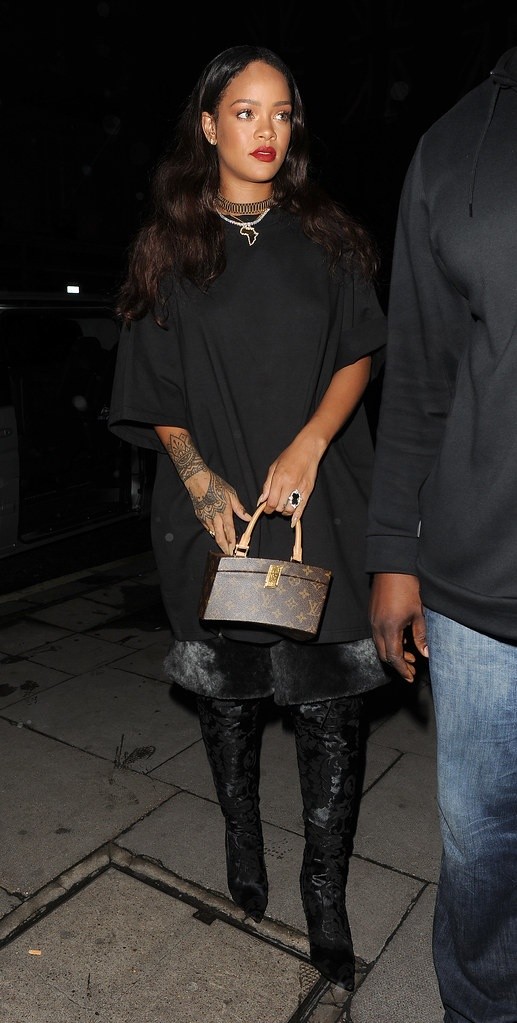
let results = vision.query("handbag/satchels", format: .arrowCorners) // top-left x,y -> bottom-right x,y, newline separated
202,501 -> 332,642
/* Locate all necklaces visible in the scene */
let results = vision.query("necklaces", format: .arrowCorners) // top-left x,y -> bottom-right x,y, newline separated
214,189 -> 278,246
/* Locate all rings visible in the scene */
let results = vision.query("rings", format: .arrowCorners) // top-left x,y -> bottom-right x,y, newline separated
289,491 -> 300,507
209,531 -> 215,536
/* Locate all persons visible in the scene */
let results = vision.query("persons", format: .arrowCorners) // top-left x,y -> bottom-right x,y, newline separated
366,39 -> 517,1023
107,44 -> 398,992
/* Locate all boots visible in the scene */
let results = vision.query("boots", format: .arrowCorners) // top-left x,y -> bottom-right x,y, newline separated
197,699 -> 268,924
290,694 -> 370,992
